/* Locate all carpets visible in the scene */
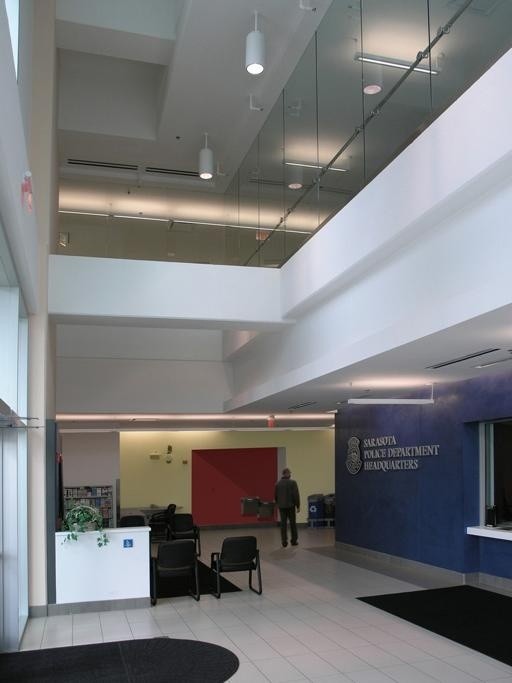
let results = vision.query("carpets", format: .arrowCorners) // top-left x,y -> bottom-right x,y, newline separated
355,584 -> 512,666
0,637 -> 239,683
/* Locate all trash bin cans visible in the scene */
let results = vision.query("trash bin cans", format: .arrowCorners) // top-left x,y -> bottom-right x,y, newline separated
307,494 -> 336,527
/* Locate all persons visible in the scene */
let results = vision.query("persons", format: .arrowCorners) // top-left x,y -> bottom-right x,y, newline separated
276,468 -> 301,547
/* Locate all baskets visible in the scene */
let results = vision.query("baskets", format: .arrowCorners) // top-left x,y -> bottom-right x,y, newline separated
65,506 -> 99,531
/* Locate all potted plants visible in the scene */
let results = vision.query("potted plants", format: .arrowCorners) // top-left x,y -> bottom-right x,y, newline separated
62,503 -> 110,548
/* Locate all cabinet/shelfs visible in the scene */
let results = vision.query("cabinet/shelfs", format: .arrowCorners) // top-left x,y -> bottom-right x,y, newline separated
63,478 -> 120,528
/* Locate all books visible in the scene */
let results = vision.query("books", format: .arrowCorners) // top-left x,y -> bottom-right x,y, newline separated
64,488 -> 111,518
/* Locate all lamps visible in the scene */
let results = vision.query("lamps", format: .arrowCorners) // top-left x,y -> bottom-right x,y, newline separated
348,381 -> 434,405
245,11 -> 265,74
199,133 -> 213,179
288,166 -> 302,189
363,61 -> 383,94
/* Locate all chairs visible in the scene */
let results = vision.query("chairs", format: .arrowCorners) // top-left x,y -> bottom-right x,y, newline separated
121,505 -> 262,605
